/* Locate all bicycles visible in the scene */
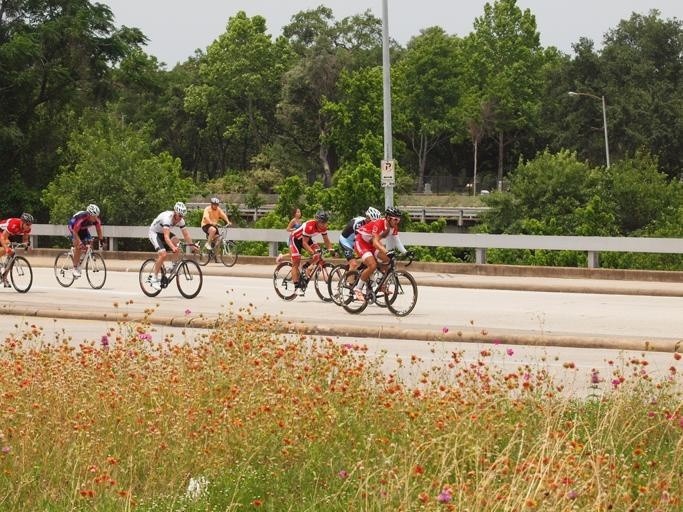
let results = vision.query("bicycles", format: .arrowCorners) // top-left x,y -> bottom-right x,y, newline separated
0,242 -> 33,293
272,247 -> 418,316
52,236 -> 108,289
137,242 -> 202,299
194,222 -> 238,267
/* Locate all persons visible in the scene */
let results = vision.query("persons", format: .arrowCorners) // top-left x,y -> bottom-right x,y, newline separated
148,201 -> 201,292
289,212 -> 332,297
352,206 -> 408,300
0,212 -> 33,289
274,208 -> 302,263
67,203 -> 104,278
201,198 -> 230,263
339,206 -> 381,294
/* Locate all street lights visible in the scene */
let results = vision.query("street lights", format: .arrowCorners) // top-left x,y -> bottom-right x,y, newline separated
466,183 -> 473,199
568,92 -> 609,167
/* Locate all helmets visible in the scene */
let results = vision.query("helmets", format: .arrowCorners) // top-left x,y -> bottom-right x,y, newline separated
86,204 -> 100,217
174,202 -> 187,216
384,207 -> 402,217
316,212 -> 330,222
210,198 -> 219,204
365,206 -> 381,218
20,213 -> 34,225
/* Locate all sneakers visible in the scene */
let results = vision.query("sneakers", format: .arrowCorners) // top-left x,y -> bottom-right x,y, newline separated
344,281 -> 364,301
276,252 -> 284,264
380,284 -> 393,295
215,254 -> 223,263
79,252 -> 86,260
72,265 -> 83,278
294,287 -> 304,296
149,275 -> 161,284
300,267 -> 308,279
169,262 -> 180,275
205,242 -> 214,250
